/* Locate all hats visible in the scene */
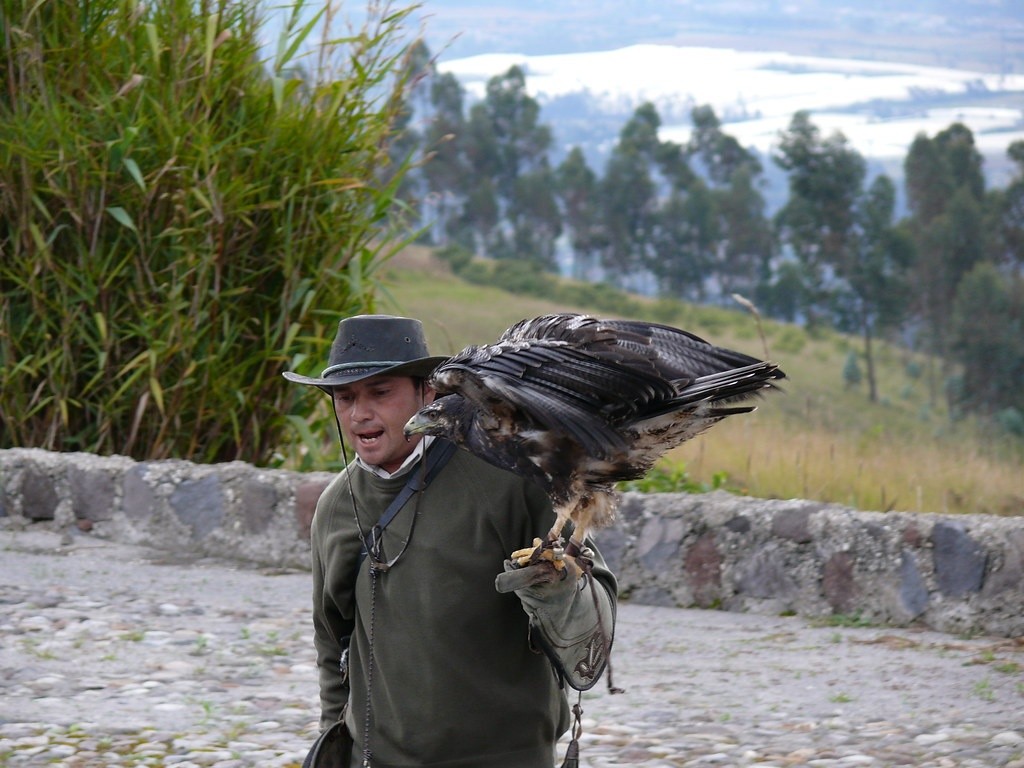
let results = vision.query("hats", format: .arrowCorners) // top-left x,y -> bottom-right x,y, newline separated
281,314 -> 452,386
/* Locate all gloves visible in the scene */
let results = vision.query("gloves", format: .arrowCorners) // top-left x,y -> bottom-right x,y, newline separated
495,548 -> 619,691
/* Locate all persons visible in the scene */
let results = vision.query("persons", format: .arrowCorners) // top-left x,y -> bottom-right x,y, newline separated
281,315 -> 618,768
